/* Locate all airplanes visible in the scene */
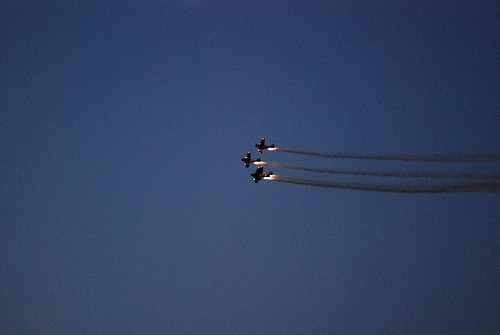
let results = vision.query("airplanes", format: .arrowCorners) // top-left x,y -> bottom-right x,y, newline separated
241,152 -> 261,168
256,137 -> 275,153
250,165 -> 273,183
